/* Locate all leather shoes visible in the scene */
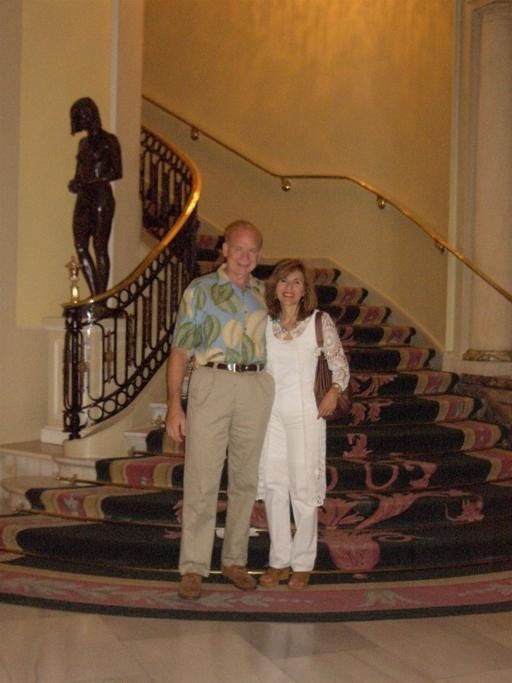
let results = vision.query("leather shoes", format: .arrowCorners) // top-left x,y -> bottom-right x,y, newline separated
223,566 -> 310,589
179,574 -> 203,600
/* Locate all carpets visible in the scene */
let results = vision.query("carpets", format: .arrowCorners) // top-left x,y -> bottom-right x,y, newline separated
1,183 -> 511,616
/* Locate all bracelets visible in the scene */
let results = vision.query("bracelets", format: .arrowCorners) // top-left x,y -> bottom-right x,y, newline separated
333,382 -> 342,398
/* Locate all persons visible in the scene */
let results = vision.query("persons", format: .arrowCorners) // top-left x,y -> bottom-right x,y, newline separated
66,95 -> 125,313
164,219 -> 274,602
256,256 -> 352,592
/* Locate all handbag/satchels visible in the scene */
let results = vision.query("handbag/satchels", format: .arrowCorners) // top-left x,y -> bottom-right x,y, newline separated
315,312 -> 352,419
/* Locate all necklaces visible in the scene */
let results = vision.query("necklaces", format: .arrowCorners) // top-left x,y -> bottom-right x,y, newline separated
276,315 -> 304,332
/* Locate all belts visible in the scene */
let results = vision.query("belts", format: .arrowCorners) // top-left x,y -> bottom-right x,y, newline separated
206,362 -> 262,371
